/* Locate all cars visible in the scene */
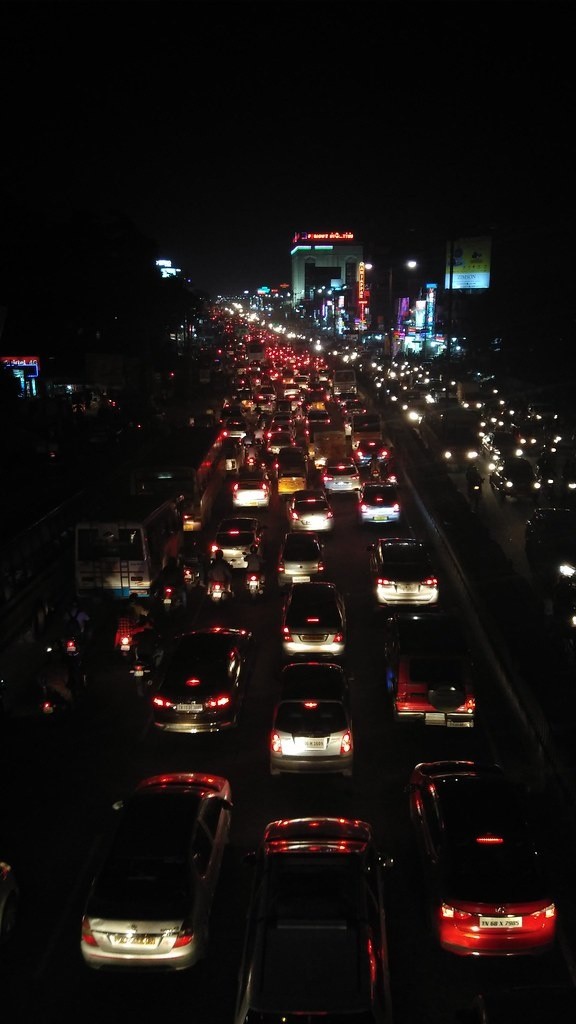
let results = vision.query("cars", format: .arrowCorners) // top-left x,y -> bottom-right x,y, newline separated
405,758 -> 558,957
0,287 -> 576,736
0,861 -> 20,935
235,816 -> 395,1024
79,771 -> 233,975
269,659 -> 354,778
